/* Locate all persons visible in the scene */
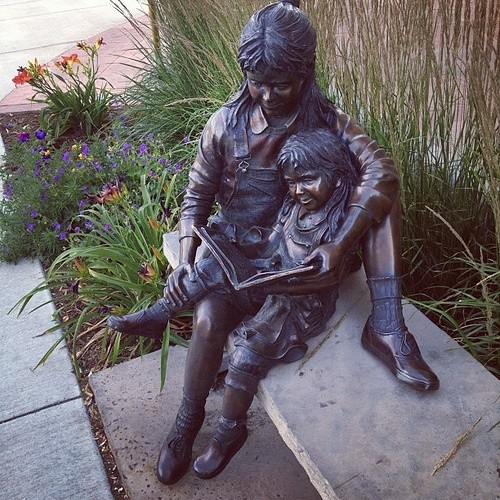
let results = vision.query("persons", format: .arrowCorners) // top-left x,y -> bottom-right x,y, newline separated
105,127 -> 365,481
156,0 -> 443,486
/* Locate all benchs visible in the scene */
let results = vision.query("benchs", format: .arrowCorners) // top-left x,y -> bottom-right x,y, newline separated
163,230 -> 500,500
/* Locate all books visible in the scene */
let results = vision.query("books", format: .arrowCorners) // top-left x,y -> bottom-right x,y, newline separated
190,223 -> 320,292
